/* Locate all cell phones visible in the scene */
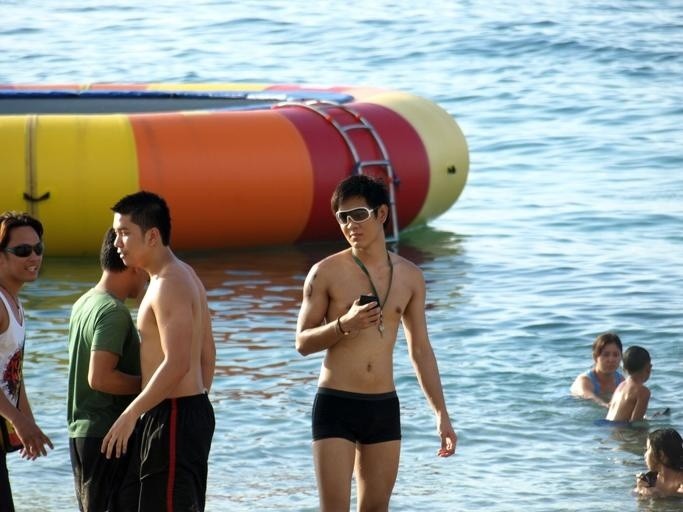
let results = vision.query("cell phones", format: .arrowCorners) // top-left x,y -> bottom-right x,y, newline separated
360,295 -> 379,308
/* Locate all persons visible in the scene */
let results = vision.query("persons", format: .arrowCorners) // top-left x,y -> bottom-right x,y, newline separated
570,333 -> 626,408
99,188 -> 220,511
293,171 -> 459,511
65,226 -> 151,512
0,208 -> 57,512
605,344 -> 671,422
631,426 -> 683,497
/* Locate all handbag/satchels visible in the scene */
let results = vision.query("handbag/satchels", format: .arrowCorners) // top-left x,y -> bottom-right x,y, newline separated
0,416 -> 24,453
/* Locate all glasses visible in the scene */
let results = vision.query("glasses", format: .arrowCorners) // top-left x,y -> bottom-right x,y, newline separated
3,242 -> 43,257
335,207 -> 374,225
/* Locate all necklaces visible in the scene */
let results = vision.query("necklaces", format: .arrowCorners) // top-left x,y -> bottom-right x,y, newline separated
0,283 -> 21,316
349,246 -> 394,336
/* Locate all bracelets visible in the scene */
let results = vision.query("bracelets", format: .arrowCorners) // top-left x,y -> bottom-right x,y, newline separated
336,314 -> 352,336
